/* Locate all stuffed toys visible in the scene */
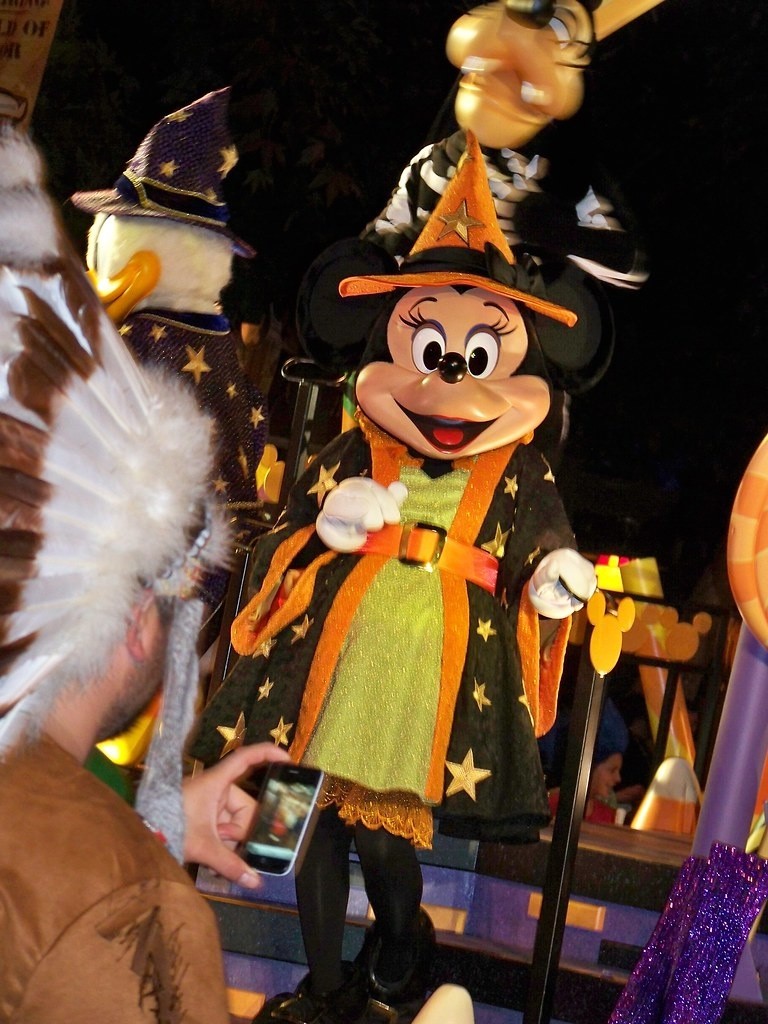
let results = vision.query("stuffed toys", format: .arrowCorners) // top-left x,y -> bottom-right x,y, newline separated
71,86 -> 262,767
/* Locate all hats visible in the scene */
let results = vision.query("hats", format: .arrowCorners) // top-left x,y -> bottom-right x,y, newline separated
0,139 -> 218,749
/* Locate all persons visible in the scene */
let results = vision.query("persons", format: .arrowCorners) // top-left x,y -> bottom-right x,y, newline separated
0,135 -> 291,1024
548,734 -> 622,828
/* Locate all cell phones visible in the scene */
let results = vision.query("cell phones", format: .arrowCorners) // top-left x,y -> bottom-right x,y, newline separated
232,759 -> 329,877
